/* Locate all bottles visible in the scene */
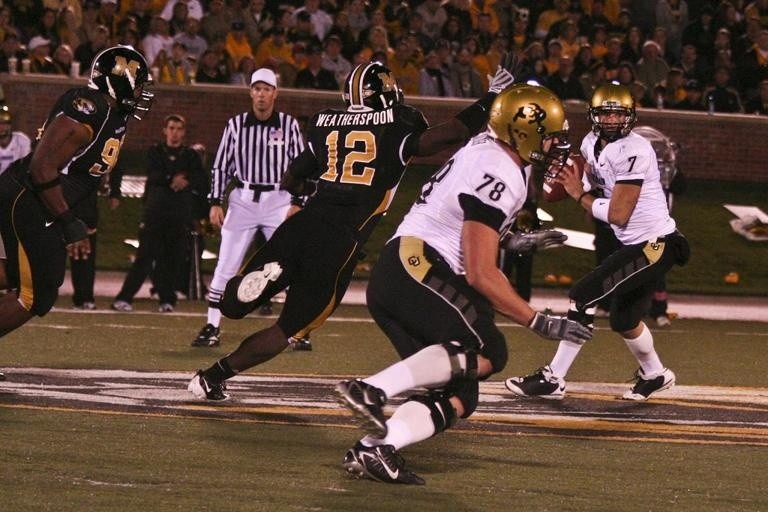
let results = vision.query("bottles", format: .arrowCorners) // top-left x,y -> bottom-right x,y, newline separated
708,95 -> 716,115
658,92 -> 664,109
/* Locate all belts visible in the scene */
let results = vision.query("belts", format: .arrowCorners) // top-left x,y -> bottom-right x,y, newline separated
233,182 -> 275,202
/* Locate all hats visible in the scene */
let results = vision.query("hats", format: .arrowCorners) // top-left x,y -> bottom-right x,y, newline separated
248,68 -> 278,89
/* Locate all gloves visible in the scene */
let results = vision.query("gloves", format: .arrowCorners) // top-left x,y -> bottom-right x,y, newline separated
532,310 -> 593,349
506,228 -> 568,255
483,60 -> 516,96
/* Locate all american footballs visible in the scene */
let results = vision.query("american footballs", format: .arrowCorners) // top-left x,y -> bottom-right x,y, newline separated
542,149 -> 586,204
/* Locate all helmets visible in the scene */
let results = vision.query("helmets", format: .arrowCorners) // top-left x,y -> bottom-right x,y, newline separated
87,44 -> 159,108
586,81 -> 637,138
488,80 -> 573,181
341,59 -> 404,113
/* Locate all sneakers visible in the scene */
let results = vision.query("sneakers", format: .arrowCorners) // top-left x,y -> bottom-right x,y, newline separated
343,442 -> 425,490
74,302 -> 96,314
503,363 -> 567,402
332,377 -> 388,441
159,304 -> 172,312
620,368 -> 676,402
190,325 -> 221,348
111,300 -> 132,311
187,367 -> 230,405
288,335 -> 314,355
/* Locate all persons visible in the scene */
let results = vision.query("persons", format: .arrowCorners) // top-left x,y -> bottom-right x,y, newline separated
0,0 -> 768,116
113,116 -> 200,311
1,103 -> 32,293
67,161 -> 123,310
504,79 -> 691,400
190,69 -> 311,351
187,61 -> 514,401
333,80 -> 593,485
593,126 -> 677,326
500,172 -> 541,302
1,45 -> 154,336
